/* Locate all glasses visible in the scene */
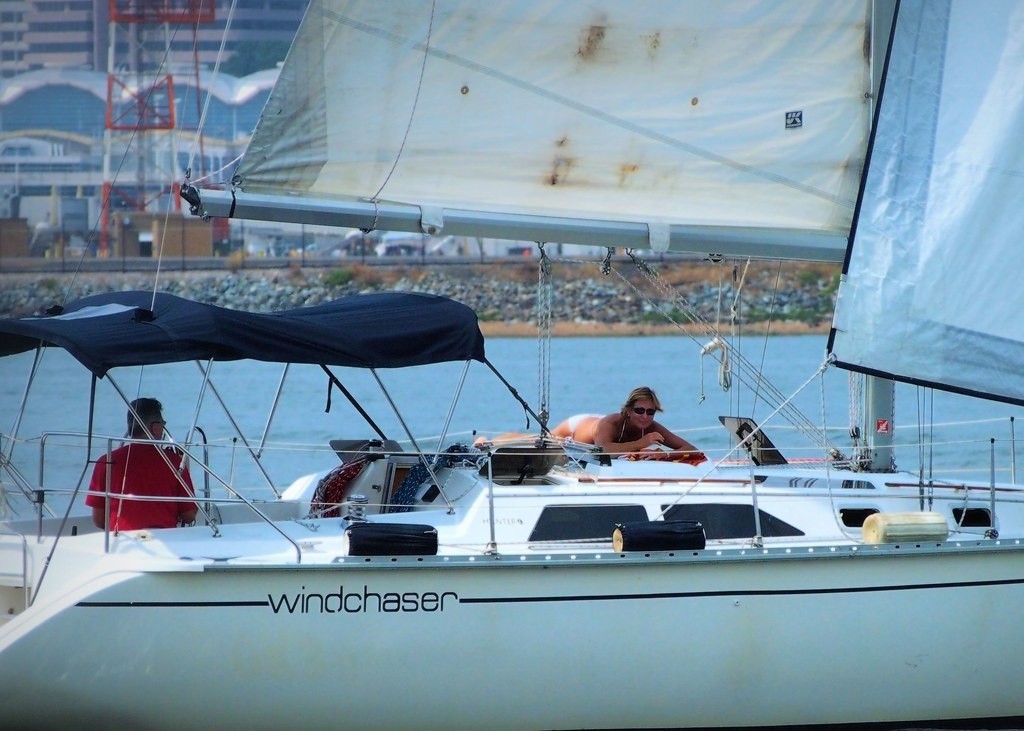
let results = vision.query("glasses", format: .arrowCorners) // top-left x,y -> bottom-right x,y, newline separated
633,407 -> 657,416
151,419 -> 167,427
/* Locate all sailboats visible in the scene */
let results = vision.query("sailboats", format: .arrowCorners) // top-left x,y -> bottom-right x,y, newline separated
0,0 -> 1024,731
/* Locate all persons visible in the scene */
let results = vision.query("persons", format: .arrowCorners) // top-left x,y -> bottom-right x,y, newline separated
86,398 -> 199,531
469,386 -> 701,461
